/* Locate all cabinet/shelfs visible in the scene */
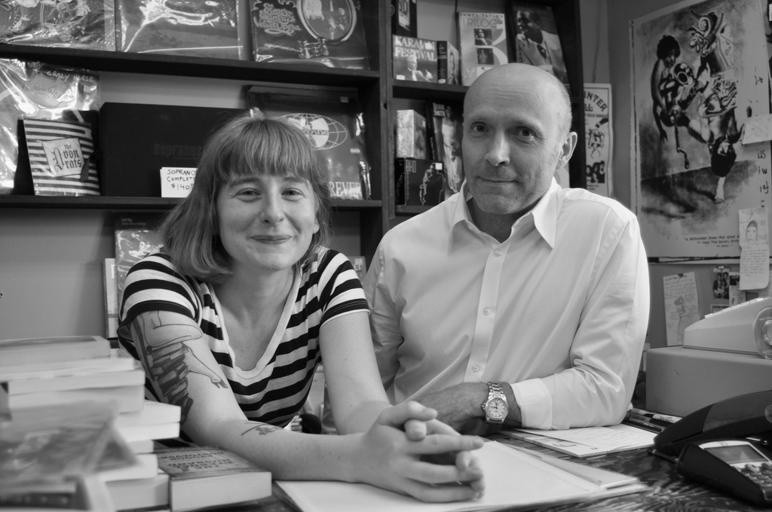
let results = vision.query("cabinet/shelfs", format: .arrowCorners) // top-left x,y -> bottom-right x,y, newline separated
386,0 -> 585,235
0,0 -> 387,274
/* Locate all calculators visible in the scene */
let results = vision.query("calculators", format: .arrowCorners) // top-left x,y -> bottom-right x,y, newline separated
677,437 -> 772,506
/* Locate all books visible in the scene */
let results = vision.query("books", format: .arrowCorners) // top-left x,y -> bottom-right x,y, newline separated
0,334 -> 277,512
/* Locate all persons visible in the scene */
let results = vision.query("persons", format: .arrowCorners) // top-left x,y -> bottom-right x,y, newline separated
474,29 -> 489,45
479,49 -> 487,63
118,110 -> 486,504
322,63 -> 649,434
516,9 -> 568,84
440,117 -> 465,202
400,51 -> 433,83
448,52 -> 459,85
744,221 -> 759,242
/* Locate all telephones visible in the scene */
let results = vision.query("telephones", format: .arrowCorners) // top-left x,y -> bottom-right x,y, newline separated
653,390 -> 772,459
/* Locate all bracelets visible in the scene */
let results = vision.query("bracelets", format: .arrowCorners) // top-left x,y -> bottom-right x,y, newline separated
481,380 -> 509,425
